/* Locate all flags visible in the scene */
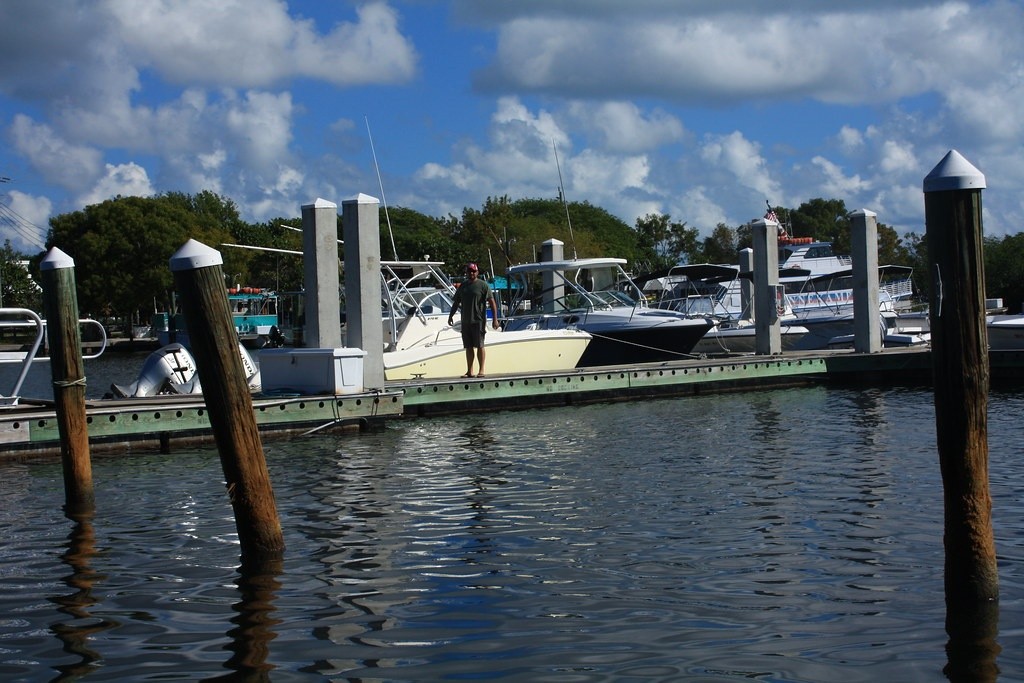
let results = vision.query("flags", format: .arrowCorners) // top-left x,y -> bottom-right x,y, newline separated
767,211 -> 785,237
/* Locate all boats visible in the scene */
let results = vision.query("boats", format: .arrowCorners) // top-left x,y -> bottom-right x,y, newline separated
108,201 -> 920,400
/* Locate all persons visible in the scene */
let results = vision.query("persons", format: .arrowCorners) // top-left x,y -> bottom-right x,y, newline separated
448,263 -> 499,378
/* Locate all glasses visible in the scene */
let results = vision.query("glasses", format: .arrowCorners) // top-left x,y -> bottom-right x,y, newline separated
467,270 -> 477,273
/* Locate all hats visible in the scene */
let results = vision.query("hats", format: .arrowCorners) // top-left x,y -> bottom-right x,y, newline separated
467,263 -> 478,271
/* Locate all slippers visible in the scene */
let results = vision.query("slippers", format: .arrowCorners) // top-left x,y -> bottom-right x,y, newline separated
477,373 -> 486,377
460,372 -> 475,378
